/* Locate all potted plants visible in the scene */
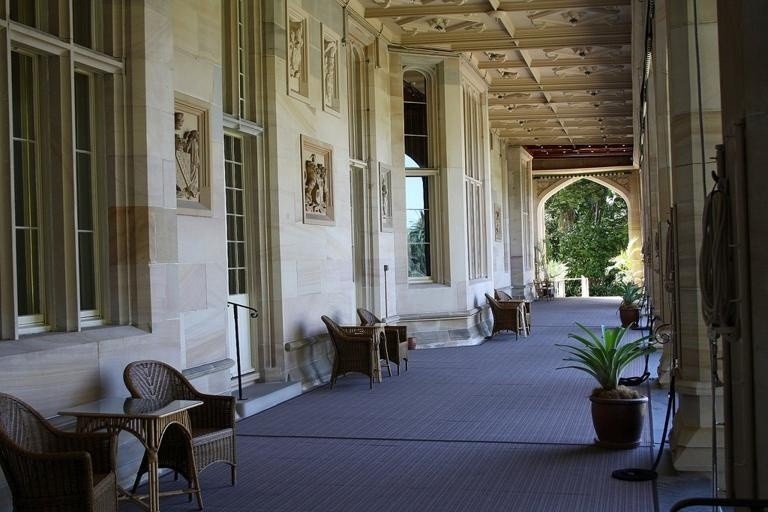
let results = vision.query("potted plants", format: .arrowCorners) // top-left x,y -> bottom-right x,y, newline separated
555,319 -> 651,451
613,281 -> 644,328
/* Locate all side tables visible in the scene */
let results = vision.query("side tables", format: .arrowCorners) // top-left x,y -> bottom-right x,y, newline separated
340,322 -> 392,384
498,298 -> 533,335
56,396 -> 208,510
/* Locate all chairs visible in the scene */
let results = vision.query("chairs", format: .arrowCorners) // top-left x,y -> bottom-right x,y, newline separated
124,360 -> 238,493
359,308 -> 408,376
495,289 -> 513,301
485,293 -> 521,340
533,279 -> 555,302
1,394 -> 119,512
322,314 -> 378,391
